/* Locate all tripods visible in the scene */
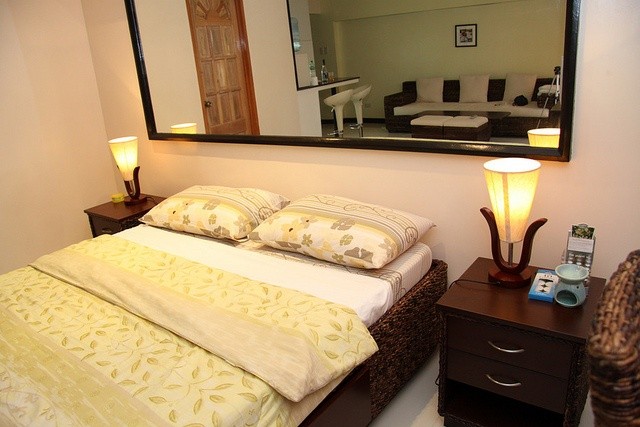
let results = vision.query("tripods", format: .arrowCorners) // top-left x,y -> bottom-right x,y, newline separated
536,67 -> 561,127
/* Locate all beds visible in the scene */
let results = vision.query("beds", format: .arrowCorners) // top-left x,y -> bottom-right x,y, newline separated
0,181 -> 448,427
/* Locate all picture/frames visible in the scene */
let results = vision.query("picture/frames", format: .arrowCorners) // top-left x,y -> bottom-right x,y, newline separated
454,23 -> 478,47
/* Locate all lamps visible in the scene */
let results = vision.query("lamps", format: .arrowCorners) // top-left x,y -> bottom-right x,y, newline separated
109,137 -> 146,202
478,158 -> 547,289
172,122 -> 199,134
527,126 -> 560,149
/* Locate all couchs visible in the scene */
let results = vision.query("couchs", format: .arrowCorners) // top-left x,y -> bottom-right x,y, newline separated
588,249 -> 640,426
383,78 -> 560,139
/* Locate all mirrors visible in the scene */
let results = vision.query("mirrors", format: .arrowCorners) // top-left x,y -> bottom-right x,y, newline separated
123,0 -> 582,163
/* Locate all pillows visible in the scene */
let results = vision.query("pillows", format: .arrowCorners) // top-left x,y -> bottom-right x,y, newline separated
459,75 -> 489,102
246,192 -> 433,270
502,71 -> 538,102
416,75 -> 445,103
137,183 -> 290,245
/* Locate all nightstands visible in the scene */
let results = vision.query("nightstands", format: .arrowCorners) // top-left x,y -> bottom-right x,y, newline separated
83,193 -> 168,238
437,254 -> 607,427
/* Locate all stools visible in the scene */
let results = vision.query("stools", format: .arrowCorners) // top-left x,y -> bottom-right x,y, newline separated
352,83 -> 373,136
323,89 -> 352,138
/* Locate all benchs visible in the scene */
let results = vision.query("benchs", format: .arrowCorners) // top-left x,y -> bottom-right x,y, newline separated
411,114 -> 490,142
